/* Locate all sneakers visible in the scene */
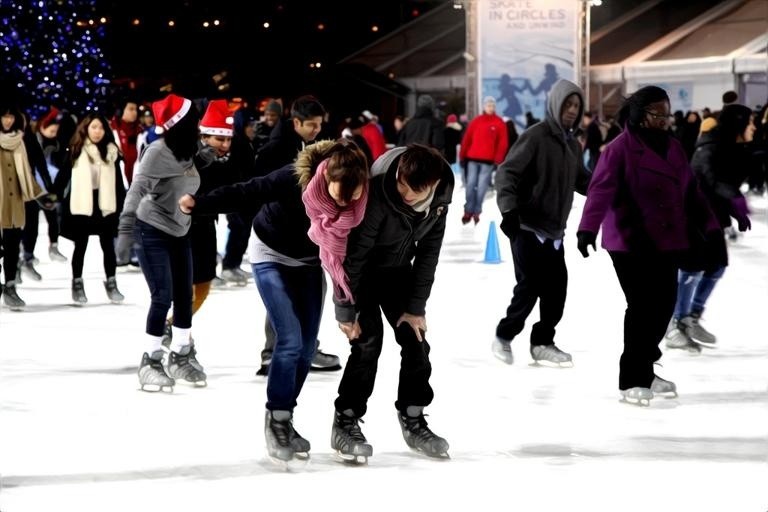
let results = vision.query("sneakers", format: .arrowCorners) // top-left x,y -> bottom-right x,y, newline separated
2,244 -> 253,307
463,210 -> 472,222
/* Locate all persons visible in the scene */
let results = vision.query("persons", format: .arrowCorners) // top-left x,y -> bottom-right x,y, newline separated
576,85 -> 751,401
331,142 -> 455,457
665,104 -> 756,353
154,98 -> 237,348
0,78 -> 767,316
491,81 -> 592,365
177,137 -> 372,462
112,93 -> 218,385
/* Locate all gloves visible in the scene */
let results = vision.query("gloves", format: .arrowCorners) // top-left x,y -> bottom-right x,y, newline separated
725,227 -> 737,243
738,213 -> 751,231
500,208 -> 520,242
199,147 -> 219,163
116,224 -> 137,266
577,231 -> 596,257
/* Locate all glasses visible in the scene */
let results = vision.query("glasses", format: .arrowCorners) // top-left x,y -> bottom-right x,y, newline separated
646,110 -> 675,122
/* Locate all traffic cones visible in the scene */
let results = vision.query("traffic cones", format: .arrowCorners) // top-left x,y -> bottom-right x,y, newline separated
477,219 -> 507,266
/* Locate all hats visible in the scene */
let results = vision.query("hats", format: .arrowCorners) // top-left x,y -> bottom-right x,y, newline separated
198,99 -> 248,137
151,94 -> 192,135
417,95 -> 434,108
